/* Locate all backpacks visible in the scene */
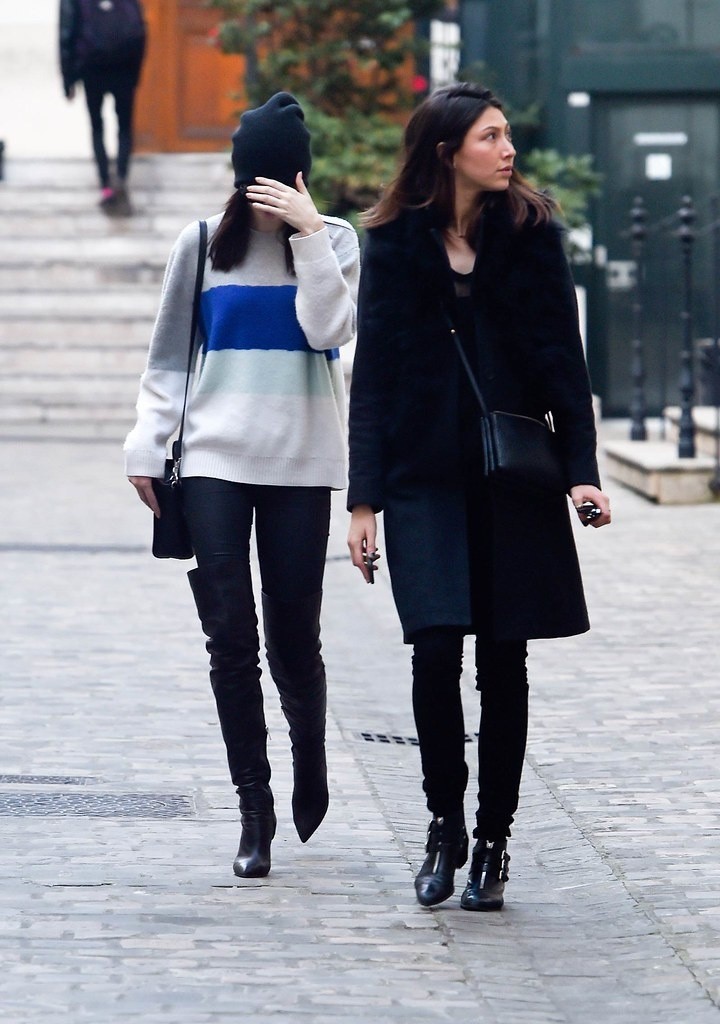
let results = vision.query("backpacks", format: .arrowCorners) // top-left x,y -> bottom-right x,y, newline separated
70,0 -> 141,66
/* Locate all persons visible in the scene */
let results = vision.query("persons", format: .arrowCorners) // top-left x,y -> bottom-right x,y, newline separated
58,0 -> 147,216
122,91 -> 361,878
346,81 -> 611,912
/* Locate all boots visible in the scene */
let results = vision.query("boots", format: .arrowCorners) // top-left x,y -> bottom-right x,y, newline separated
259,588 -> 330,843
188,563 -> 277,878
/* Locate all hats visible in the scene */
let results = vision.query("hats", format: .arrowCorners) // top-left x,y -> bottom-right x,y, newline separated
231,91 -> 312,189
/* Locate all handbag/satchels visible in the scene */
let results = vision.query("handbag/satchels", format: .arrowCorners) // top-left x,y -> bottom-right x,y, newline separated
151,440 -> 194,560
479,405 -> 564,501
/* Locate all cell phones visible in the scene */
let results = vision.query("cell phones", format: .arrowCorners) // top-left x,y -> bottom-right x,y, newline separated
367,557 -> 376,584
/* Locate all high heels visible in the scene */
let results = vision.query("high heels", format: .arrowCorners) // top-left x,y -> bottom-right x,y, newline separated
460,839 -> 511,912
414,814 -> 469,907
